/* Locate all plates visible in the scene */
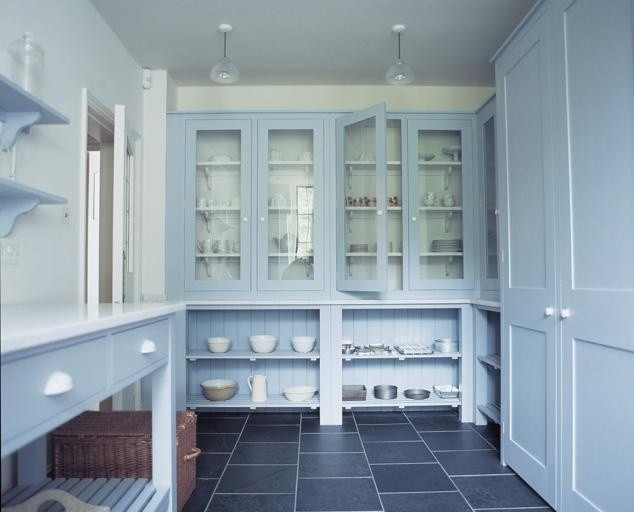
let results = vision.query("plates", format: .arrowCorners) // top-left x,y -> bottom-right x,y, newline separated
375,241 -> 394,253
431,238 -> 464,253
349,242 -> 369,254
399,240 -> 404,253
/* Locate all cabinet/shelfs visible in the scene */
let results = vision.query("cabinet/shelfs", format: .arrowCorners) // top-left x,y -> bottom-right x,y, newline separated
489,0 -> 634,512
0,70 -> 71,239
330,100 -> 477,299
474,306 -> 500,429
475,82 -> 500,305
164,110 -> 334,301
179,304 -> 330,426
330,303 -> 475,427
2,300 -> 185,511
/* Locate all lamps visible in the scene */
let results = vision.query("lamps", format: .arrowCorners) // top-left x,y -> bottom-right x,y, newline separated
207,22 -> 242,87
386,24 -> 414,86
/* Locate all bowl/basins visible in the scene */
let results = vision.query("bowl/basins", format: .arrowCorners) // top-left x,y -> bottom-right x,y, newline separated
433,338 -> 459,354
200,378 -> 239,402
374,385 -> 398,399
441,192 -> 456,207
205,336 -> 232,353
281,385 -> 319,402
404,388 -> 432,400
247,334 -> 280,354
290,335 -> 316,352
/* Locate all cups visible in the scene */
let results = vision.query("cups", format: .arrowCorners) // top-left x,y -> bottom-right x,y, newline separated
269,146 -> 283,161
196,197 -> 240,208
422,190 -> 437,208
196,238 -> 239,254
299,151 -> 312,161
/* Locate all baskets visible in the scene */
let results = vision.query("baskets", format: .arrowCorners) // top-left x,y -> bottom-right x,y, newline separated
53,415 -> 201,510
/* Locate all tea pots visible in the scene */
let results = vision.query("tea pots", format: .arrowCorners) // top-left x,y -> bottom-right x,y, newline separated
246,373 -> 269,402
272,231 -> 300,253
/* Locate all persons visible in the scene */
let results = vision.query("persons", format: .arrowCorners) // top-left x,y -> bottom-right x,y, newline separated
281,248 -> 314,280
